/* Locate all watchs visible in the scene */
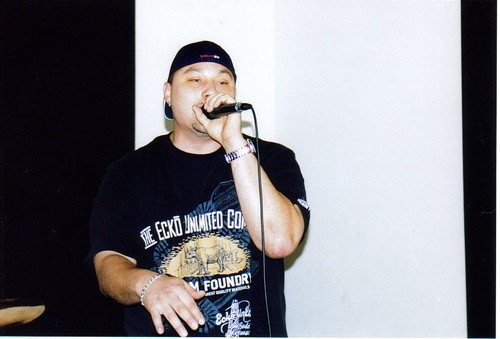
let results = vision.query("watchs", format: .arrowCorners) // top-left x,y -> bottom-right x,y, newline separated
224,137 -> 258,164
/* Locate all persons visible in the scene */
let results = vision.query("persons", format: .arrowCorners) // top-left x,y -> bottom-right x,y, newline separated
88,40 -> 313,337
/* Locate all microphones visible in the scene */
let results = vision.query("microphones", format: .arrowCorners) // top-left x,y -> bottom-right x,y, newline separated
202,103 -> 252,119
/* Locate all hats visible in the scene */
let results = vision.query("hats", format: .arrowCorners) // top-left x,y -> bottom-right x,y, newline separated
163,40 -> 237,121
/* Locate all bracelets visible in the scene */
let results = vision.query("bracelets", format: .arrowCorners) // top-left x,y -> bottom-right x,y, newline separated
140,269 -> 168,308
